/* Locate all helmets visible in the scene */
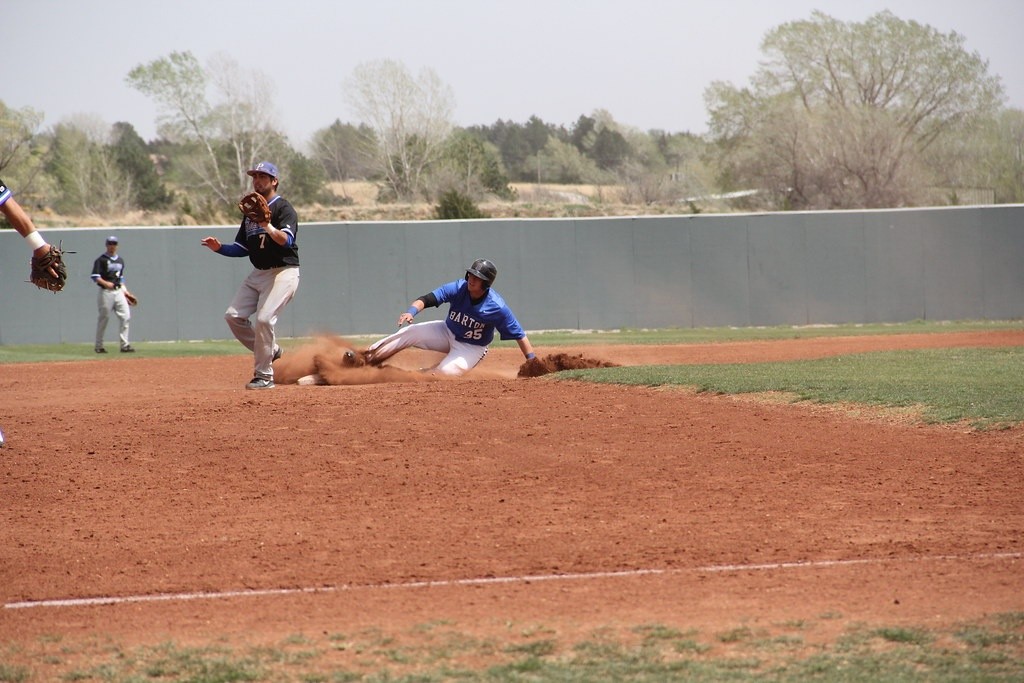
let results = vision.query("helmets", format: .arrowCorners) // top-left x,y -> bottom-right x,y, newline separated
465,259 -> 497,289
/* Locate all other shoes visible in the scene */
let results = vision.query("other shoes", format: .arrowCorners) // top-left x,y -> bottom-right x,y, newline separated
121,344 -> 133,351
95,348 -> 108,353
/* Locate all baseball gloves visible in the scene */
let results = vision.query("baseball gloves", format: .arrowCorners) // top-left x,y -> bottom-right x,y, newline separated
30,243 -> 67,292
125,294 -> 137,305
238,193 -> 271,224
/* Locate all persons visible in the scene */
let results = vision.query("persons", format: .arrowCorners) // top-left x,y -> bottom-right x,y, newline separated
200,162 -> 299,390
343,257 -> 535,378
0,178 -> 62,279
91,236 -> 138,353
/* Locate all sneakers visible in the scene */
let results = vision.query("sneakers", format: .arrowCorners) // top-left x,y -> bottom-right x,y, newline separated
272,347 -> 283,362
343,352 -> 363,367
245,378 -> 274,390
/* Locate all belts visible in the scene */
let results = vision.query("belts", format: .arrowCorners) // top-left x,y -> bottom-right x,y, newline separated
257,264 -> 287,270
113,286 -> 121,290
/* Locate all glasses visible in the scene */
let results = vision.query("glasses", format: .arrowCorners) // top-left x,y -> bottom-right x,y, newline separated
108,242 -> 117,246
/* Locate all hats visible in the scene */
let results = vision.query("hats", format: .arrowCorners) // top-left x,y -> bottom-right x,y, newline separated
106,236 -> 117,243
246,162 -> 279,178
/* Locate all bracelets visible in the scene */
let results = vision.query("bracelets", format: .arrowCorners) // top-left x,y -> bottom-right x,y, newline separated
263,223 -> 276,234
525,352 -> 534,359
406,306 -> 419,317
24,231 -> 47,250
